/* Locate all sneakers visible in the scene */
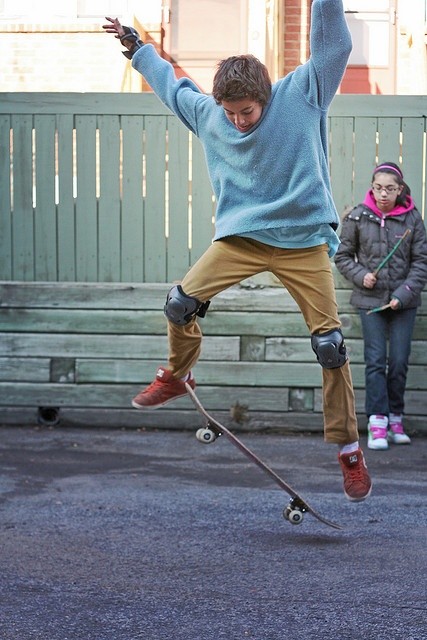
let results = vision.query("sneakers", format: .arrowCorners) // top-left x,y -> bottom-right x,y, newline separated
338,447 -> 372,502
387,412 -> 411,445
131,366 -> 195,411
367,415 -> 388,450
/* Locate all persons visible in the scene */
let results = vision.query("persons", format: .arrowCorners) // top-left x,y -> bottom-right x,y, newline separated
333,162 -> 427,451
103,0 -> 373,503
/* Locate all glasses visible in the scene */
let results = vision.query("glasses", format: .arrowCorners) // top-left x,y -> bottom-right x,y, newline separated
371,185 -> 399,193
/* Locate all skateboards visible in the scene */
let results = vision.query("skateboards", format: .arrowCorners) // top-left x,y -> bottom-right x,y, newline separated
184,382 -> 344,530
